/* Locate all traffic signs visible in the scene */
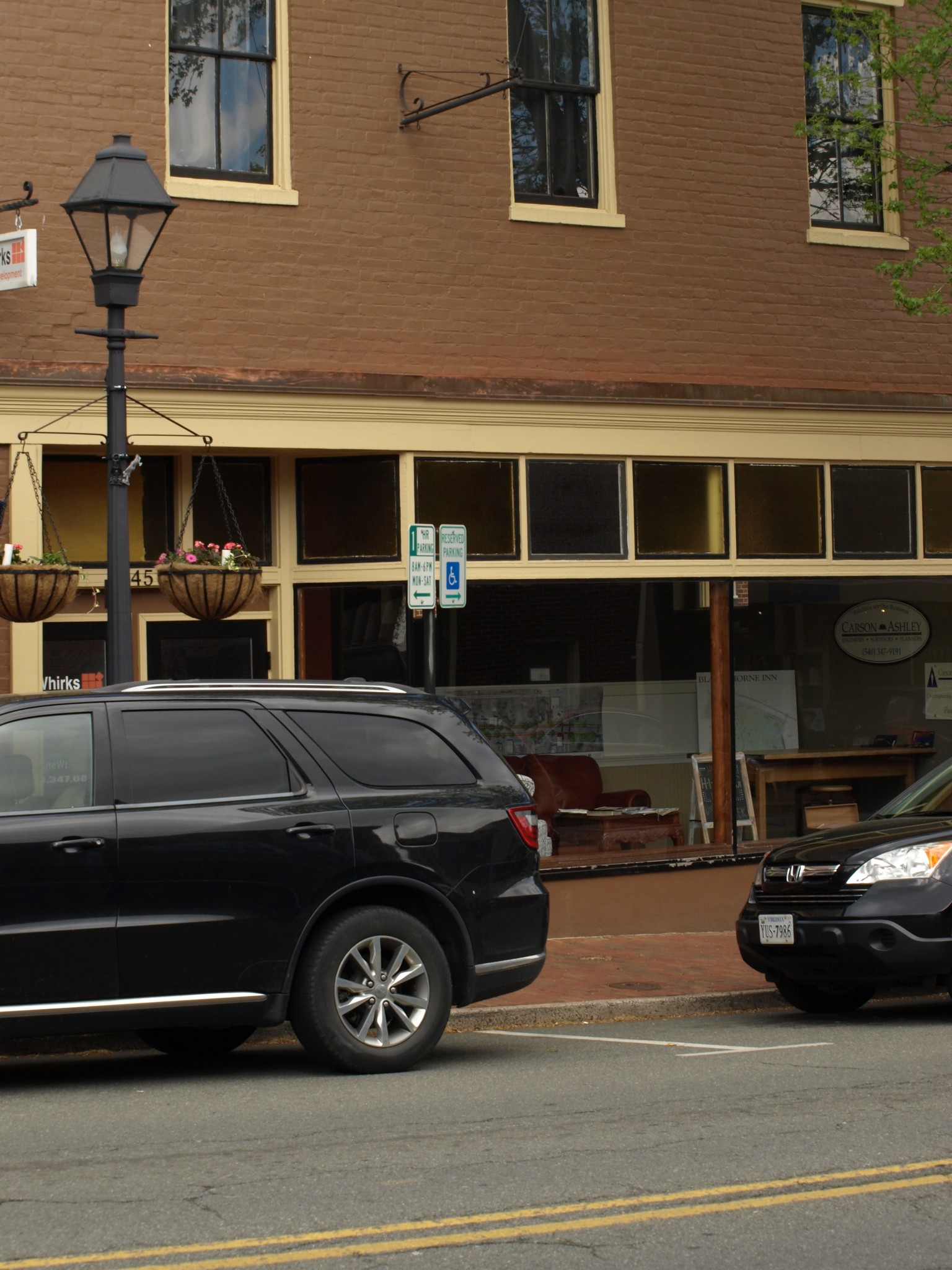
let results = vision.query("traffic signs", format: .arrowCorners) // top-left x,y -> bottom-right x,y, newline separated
407,523 -> 436,609
438,524 -> 467,609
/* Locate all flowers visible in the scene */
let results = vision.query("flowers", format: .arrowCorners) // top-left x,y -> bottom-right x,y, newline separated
157,538 -> 258,570
0,543 -> 78,566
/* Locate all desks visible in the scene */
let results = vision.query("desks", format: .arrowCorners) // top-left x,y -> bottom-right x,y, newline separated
741,742 -> 938,842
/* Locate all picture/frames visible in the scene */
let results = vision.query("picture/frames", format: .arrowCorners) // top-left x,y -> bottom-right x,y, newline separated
832,597 -> 932,665
910,730 -> 936,748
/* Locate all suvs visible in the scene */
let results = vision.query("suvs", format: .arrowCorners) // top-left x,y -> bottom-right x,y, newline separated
0,678 -> 551,1076
735,757 -> 952,1013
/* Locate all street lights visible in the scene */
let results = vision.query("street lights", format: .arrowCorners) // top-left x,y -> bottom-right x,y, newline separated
57,134 -> 180,688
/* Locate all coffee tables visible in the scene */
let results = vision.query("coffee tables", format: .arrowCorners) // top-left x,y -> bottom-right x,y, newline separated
549,804 -> 686,856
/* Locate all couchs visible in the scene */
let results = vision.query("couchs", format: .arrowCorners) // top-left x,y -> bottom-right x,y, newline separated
501,750 -> 653,855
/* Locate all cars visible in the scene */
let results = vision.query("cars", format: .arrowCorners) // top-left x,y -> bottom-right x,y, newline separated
490,704 -> 776,829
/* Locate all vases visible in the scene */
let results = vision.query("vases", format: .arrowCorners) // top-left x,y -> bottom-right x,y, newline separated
151,561 -> 263,620
0,563 -> 80,624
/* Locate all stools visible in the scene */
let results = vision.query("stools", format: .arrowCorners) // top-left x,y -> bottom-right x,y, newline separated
811,781 -> 853,807
